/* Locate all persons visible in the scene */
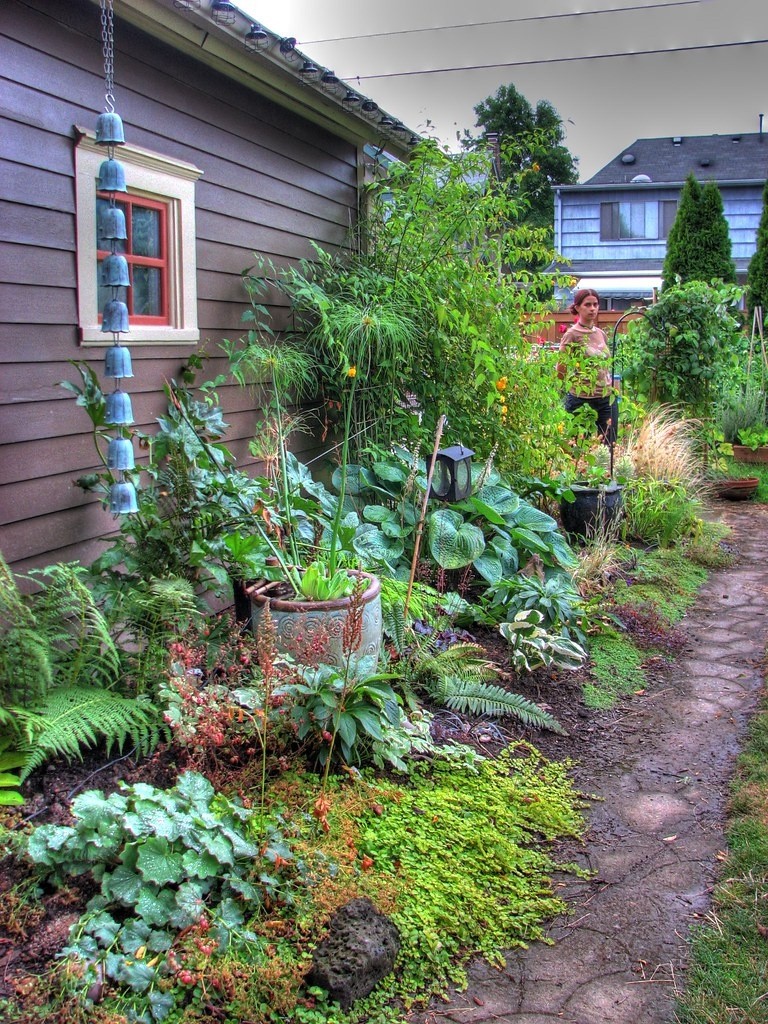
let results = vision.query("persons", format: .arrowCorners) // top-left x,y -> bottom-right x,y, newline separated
558,289 -> 618,445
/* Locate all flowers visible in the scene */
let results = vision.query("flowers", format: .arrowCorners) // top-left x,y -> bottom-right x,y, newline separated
163,294 -> 424,601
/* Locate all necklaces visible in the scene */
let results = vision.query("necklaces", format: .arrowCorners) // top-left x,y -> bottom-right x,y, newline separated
578,321 -> 594,330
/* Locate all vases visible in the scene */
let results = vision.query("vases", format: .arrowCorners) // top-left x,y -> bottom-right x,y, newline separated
250,568 -> 381,682
561,481 -> 625,542
228,565 -> 297,634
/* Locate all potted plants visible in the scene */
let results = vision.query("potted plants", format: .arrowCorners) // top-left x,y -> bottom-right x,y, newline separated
716,457 -> 760,499
717,388 -> 768,465
